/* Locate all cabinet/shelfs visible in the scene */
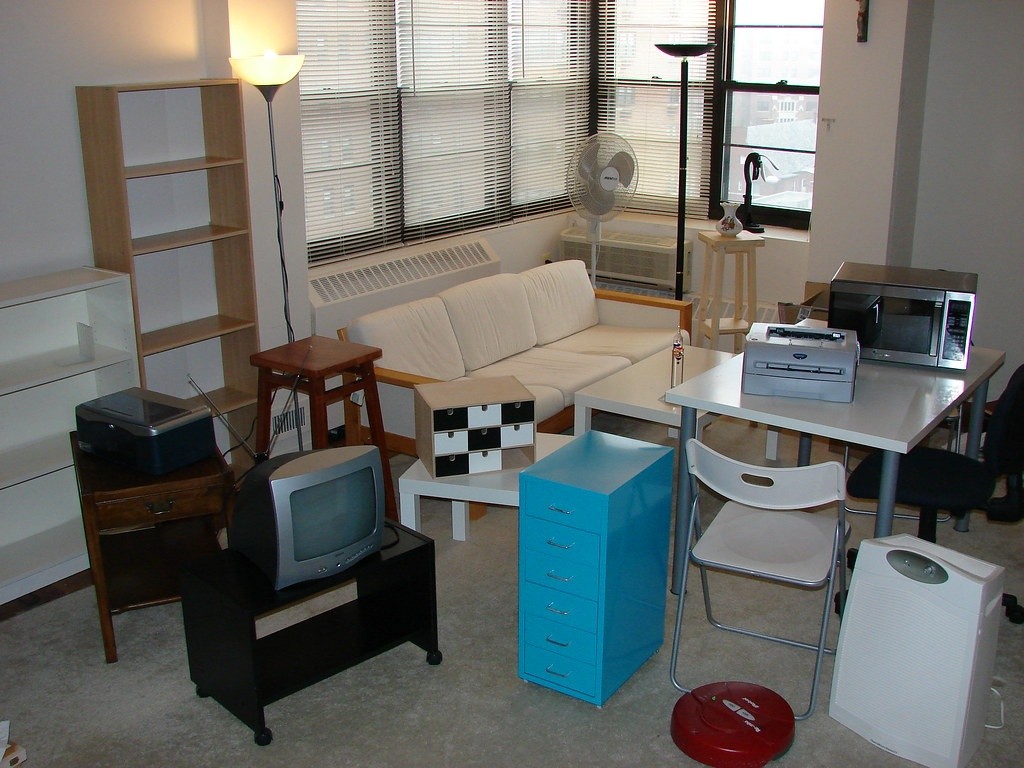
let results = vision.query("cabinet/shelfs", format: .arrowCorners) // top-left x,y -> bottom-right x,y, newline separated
518,430 -> 675,710
414,375 -> 537,480
0,264 -> 145,619
75,77 -> 262,492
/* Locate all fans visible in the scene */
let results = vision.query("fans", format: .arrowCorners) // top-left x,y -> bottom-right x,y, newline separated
566,132 -> 639,291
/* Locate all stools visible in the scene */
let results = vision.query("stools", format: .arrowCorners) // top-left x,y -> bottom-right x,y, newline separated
250,335 -> 398,522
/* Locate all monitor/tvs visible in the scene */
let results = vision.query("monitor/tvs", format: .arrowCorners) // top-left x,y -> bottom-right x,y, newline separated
185,347 -> 386,591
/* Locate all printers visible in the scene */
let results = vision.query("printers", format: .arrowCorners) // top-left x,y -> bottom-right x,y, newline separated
742,322 -> 858,403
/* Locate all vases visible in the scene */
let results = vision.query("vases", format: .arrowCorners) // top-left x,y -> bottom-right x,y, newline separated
717,202 -> 743,238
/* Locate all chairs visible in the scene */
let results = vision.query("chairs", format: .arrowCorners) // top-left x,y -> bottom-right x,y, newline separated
670,438 -> 851,720
847,364 -> 1024,624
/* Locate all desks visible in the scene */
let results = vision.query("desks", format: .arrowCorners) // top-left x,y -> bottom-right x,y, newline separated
398,432 -> 579,542
69,431 -> 234,664
665,317 -> 1006,595
181,517 -> 442,746
574,345 -> 739,436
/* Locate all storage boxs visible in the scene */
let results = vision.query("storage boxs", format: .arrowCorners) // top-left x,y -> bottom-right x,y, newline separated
778,281 -> 831,324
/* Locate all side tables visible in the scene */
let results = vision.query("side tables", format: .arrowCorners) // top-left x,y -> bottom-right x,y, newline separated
697,231 -> 765,428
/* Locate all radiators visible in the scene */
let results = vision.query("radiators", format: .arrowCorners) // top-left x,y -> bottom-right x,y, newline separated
307,238 -> 503,429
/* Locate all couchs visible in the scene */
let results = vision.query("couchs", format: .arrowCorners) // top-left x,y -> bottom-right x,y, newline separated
335,260 -> 692,523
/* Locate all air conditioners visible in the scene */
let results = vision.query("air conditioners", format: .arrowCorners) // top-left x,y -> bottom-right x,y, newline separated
560,228 -> 694,294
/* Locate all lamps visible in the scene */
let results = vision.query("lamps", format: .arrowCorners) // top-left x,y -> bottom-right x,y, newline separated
654,43 -> 719,300
227,54 -> 306,451
